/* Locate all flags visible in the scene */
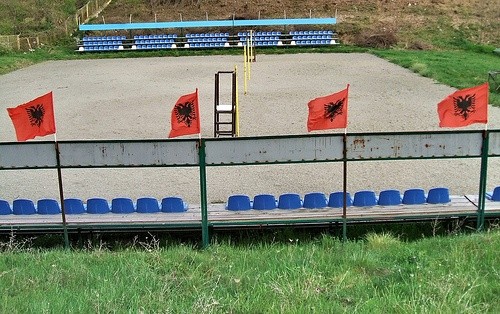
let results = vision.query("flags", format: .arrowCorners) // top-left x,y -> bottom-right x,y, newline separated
7,91 -> 55,141
307,89 -> 348,131
168,91 -> 200,138
438,82 -> 489,127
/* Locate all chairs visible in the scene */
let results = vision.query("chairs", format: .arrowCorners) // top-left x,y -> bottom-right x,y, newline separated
484,185 -> 500,203
225,187 -> 452,212
82,28 -> 333,52
0,198 -> 189,215
